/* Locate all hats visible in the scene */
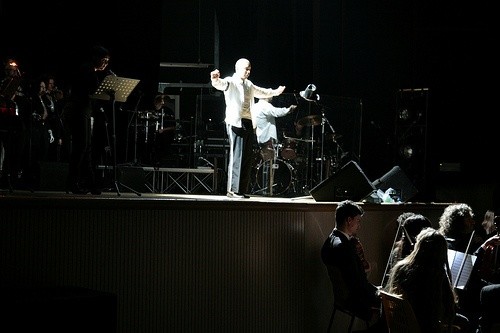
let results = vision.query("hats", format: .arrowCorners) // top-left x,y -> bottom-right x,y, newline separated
299,83 -> 321,103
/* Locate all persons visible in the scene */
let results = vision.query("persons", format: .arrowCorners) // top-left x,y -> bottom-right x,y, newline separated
210,58 -> 286,199
0,45 -> 177,195
249,98 -> 297,163
322,200 -> 500,333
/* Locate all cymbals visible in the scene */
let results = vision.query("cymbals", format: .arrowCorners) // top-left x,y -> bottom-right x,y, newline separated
298,114 -> 324,126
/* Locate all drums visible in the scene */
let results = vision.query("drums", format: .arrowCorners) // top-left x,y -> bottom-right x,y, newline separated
326,133 -> 351,160
255,158 -> 292,195
280,139 -> 297,160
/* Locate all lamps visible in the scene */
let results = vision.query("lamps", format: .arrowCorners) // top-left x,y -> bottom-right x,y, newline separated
8,59 -> 19,72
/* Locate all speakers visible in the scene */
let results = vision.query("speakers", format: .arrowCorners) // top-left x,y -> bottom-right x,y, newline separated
372,166 -> 420,202
309,160 -> 378,202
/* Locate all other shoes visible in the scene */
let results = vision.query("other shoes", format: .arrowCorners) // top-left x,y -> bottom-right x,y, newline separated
226,192 -> 244,198
235,193 -> 250,198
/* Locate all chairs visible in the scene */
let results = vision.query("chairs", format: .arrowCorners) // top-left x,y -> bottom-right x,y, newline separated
380,293 -> 452,333
324,262 -> 381,332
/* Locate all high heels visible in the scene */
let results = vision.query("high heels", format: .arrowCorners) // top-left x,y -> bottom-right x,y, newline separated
63,181 -> 88,195
83,182 -> 101,195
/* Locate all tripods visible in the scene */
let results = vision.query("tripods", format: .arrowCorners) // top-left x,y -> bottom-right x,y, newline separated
89,75 -> 141,197
